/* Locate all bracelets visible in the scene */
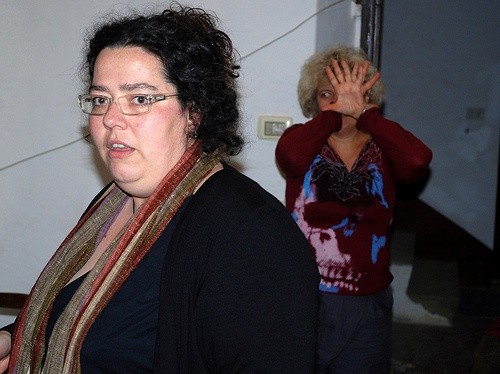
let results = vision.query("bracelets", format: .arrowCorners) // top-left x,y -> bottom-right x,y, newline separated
362,103 -> 368,113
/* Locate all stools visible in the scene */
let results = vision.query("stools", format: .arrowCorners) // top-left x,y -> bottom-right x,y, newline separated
473,315 -> 500,362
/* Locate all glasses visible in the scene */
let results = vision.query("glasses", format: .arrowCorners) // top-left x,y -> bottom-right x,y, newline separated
78,93 -> 183,116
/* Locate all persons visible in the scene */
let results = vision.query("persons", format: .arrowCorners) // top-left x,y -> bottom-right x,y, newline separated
275,42 -> 433,374
0,1 -> 320,374
